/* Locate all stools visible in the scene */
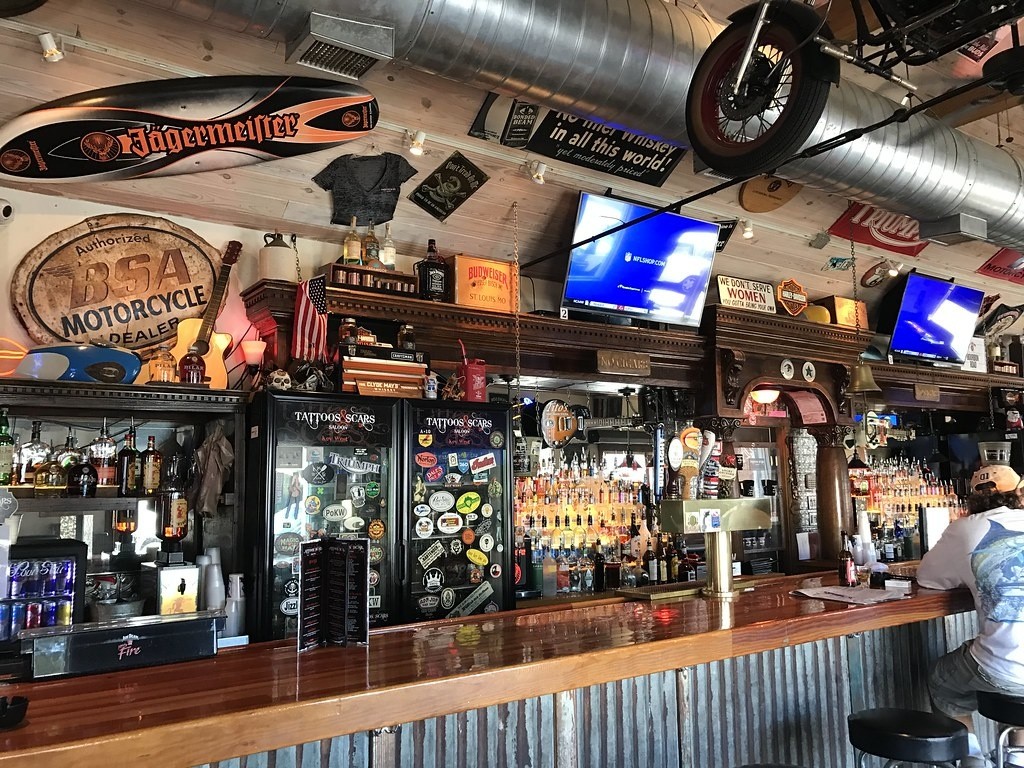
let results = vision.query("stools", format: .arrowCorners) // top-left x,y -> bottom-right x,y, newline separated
848,709 -> 968,768
975,690 -> 1024,768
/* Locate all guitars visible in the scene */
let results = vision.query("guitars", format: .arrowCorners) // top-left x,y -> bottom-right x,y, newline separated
164,239 -> 245,390
539,398 -> 645,449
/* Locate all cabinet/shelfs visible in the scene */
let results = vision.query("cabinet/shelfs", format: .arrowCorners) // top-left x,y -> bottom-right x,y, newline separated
0,377 -> 248,668
252,387 -> 515,644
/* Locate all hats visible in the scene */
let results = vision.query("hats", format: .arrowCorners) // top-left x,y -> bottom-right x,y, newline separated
971,464 -> 1024,493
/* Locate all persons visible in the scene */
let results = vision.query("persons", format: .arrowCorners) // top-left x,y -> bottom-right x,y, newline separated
916,463 -> 1024,768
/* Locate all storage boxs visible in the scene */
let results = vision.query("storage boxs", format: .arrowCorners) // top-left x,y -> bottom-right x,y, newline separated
813,294 -> 869,329
140,561 -> 200,615
445,255 -> 520,313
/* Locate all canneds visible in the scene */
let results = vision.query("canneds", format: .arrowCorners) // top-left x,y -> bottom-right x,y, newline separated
0,560 -> 72,643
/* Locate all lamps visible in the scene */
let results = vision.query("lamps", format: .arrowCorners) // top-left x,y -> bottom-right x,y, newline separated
738,219 -> 753,238
524,160 -> 547,184
37,31 -> 65,61
887,259 -> 903,276
405,129 -> 426,155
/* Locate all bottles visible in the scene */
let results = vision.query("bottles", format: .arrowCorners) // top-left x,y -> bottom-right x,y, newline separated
177,346 -> 207,385
397,325 -> 416,351
849,453 -> 971,563
0,407 -> 162,498
379,222 -> 396,272
987,334 -> 1007,362
418,239 -> 450,303
338,318 -> 359,345
154,484 -> 188,542
512,450 -> 693,598
344,216 -> 361,266
148,345 -> 178,383
361,218 -> 380,268
838,530 -> 859,588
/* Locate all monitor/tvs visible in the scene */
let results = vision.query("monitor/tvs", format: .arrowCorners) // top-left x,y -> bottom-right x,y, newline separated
559,190 -> 721,327
887,272 -> 985,366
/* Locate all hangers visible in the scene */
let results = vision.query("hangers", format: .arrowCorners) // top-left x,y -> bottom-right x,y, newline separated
349,131 -> 383,161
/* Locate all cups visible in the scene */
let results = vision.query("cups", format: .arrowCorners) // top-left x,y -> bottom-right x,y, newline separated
195,547 -> 246,638
